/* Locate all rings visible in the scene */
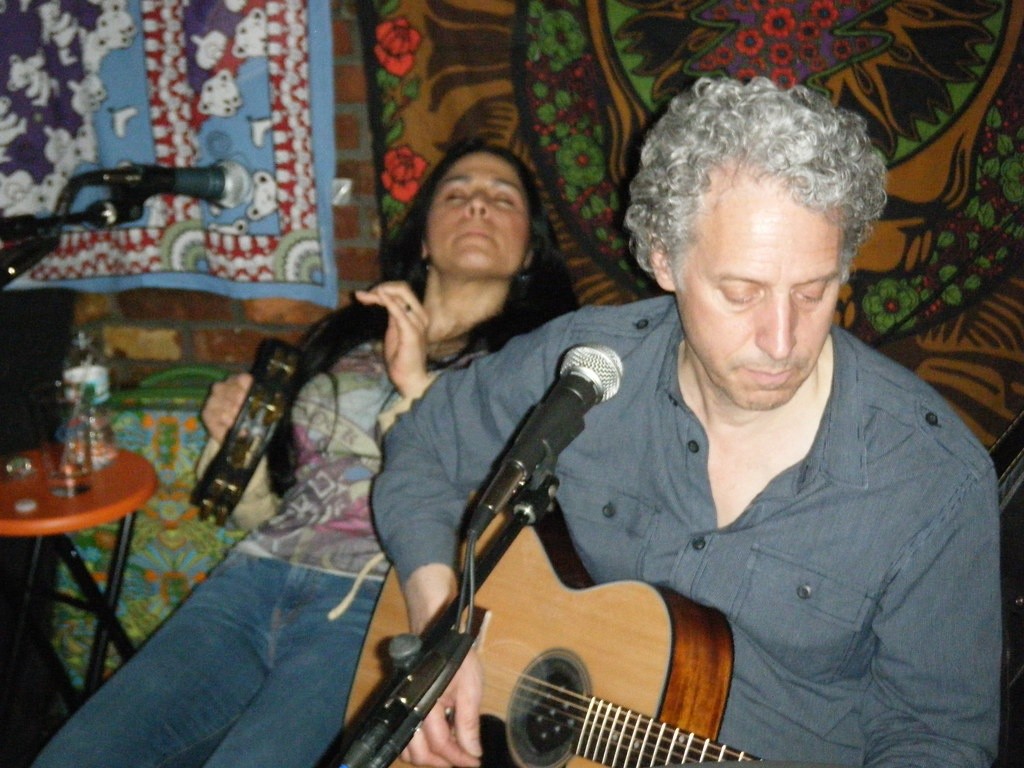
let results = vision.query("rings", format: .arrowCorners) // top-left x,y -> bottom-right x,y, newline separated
405,304 -> 410,313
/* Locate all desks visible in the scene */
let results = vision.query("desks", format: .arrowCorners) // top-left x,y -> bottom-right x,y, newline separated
0,441 -> 157,728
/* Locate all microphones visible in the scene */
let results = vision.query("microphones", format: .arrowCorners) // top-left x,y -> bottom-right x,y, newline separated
468,343 -> 623,539
77,161 -> 255,209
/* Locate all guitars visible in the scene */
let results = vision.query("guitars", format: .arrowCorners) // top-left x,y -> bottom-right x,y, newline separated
340,483 -> 773,768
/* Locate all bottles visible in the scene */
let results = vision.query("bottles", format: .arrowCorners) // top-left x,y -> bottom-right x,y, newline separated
63,327 -> 115,465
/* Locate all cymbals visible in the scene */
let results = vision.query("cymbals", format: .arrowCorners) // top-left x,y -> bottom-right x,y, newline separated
185,334 -> 310,530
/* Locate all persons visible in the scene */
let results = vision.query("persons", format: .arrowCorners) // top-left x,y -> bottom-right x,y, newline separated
370,76 -> 1004,768
27,140 -> 581,767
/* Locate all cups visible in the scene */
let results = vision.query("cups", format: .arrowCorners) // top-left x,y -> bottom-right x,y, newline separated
34,383 -> 93,498
